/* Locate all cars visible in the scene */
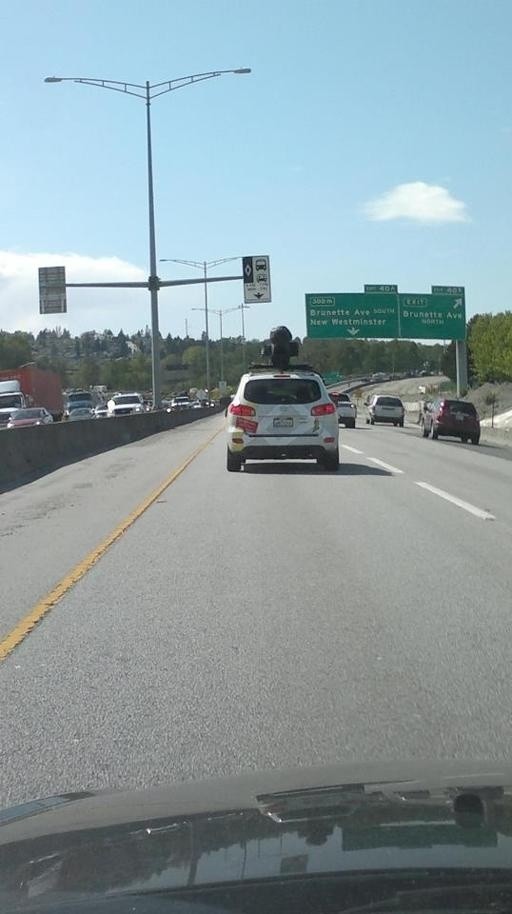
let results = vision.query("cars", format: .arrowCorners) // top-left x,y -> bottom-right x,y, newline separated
0,367 -> 221,429
365,396 -> 481,444
219,326 -> 357,471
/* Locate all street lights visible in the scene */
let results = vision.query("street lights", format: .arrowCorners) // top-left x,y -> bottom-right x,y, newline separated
159,257 -> 250,400
44,68 -> 251,409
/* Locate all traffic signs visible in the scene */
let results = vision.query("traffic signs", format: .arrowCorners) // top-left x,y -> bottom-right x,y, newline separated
243,255 -> 271,303
305,285 -> 465,338
